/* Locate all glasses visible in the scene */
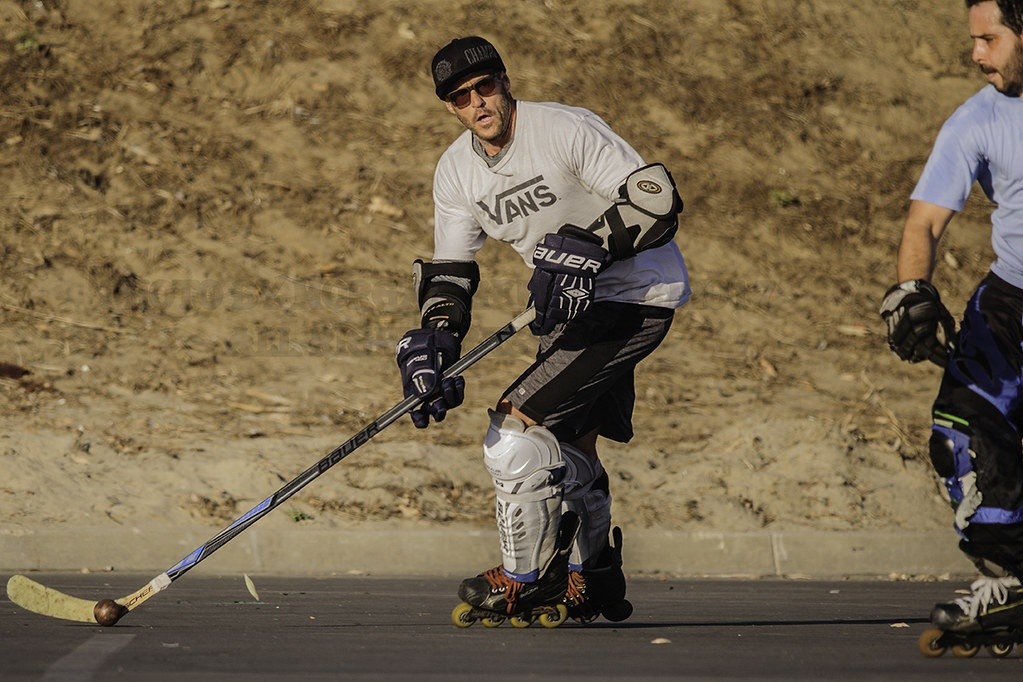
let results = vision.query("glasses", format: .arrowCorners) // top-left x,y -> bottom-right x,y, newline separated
444,72 -> 502,107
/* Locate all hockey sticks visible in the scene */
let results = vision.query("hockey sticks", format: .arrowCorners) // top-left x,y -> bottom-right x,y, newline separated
6,303 -> 535,623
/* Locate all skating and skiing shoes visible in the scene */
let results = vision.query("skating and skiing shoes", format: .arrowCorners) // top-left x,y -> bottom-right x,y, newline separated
450,510 -> 583,629
918,539 -> 1023,656
562,526 -> 633,622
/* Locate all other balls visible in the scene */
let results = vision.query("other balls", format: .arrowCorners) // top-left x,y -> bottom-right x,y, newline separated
94,599 -> 120,626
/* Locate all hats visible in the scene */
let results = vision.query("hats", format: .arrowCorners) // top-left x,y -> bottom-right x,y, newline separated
431,35 -> 506,98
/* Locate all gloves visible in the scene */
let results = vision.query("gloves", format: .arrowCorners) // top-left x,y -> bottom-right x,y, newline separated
526,225 -> 610,335
396,320 -> 465,429
878,278 -> 957,363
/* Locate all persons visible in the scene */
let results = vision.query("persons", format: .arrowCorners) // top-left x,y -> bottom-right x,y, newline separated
396,35 -> 694,628
881,0 -> 1023,660
